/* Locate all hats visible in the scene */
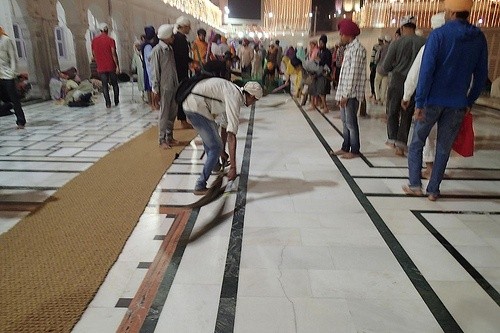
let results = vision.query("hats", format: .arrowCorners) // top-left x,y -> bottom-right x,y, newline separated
444,0 -> 472,11
320,34 -> 327,43
244,80 -> 263,100
378,33 -> 385,40
99,22 -> 107,30
290,56 -> 298,67
338,19 -> 360,38
430,12 -> 445,30
385,34 -> 392,42
400,16 -> 416,27
176,16 -> 191,27
157,24 -> 174,39
145,26 -> 155,38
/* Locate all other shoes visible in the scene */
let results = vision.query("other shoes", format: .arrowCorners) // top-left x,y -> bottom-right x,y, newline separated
17,124 -> 24,129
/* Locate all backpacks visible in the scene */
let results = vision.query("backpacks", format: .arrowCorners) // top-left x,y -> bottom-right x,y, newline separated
174,72 -> 213,103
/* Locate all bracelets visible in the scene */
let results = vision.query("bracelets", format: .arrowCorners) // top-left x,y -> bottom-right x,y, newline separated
230,166 -> 236,169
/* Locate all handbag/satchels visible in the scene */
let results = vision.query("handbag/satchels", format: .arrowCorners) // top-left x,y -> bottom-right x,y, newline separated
453,111 -> 475,157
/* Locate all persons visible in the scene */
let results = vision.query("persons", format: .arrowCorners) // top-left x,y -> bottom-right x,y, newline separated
182,77 -> 263,196
90,22 -> 120,109
149,24 -> 184,150
0,27 -> 28,128
191,28 -> 346,115
402,0 -> 489,202
172,16 -> 192,129
382,20 -> 426,156
402,11 -> 446,180
370,29 -> 401,107
329,19 -> 368,159
131,25 -> 160,110
49,67 -> 82,101
